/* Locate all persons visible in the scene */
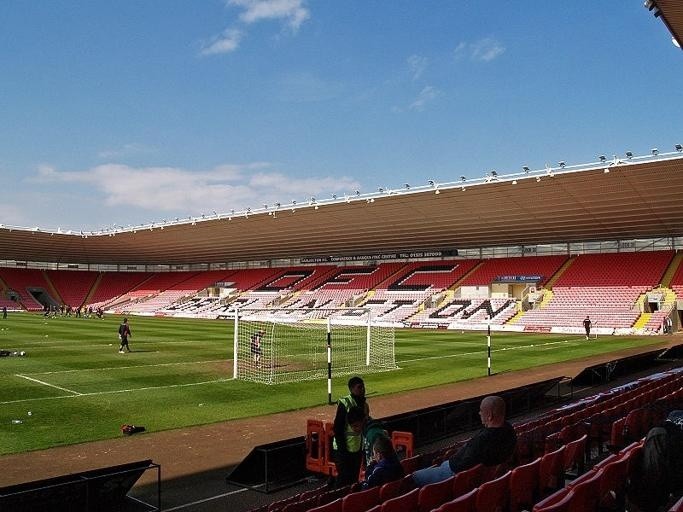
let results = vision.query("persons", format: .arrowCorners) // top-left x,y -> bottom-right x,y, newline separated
3,306 -> 7,319
351,437 -> 404,493
583,316 -> 592,340
251,330 -> 266,369
663,316 -> 667,333
118,318 -> 132,353
347,407 -> 392,467
332,377 -> 369,488
666,316 -> 673,334
411,396 -> 516,485
44,301 -> 104,320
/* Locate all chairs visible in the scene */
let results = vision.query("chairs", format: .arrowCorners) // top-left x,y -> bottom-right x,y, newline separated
248,369 -> 683,512
